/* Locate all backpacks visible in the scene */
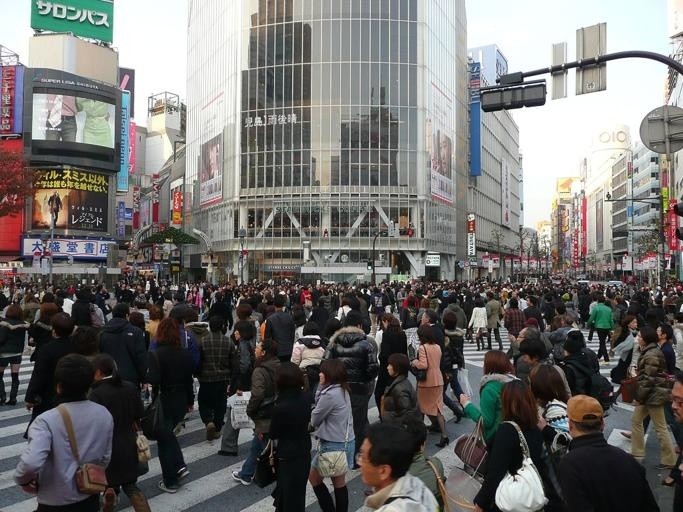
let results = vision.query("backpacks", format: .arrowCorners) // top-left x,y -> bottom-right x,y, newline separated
564,360 -> 613,411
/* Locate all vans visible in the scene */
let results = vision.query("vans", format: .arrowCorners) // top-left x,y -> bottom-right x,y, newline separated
522,278 -> 540,291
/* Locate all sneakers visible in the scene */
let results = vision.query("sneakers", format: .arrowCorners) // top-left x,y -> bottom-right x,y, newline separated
176,466 -> 190,482
104,488 -> 115,512
158,480 -> 177,493
218,450 -> 236,456
620,431 -> 682,486
206,423 -> 221,441
231,470 -> 251,486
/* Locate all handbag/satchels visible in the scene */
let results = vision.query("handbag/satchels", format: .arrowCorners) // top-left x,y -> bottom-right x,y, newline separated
132,421 -> 152,464
622,377 -> 635,403
371,307 -> 376,312
410,364 -> 426,381
142,392 -> 165,440
316,450 -> 349,478
427,414 -> 549,512
77,462 -> 109,495
252,439 -> 278,488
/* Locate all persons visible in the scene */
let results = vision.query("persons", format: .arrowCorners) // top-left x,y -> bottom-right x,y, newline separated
431,128 -> 453,182
76,99 -> 111,149
2,267 -> 682,511
43,94 -> 78,142
202,133 -> 222,200
47,189 -> 64,229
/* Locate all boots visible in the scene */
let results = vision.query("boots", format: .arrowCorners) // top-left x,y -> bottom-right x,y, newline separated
333,486 -> 348,512
0,372 -> 6,405
6,373 -> 19,405
313,483 -> 335,512
130,490 -> 150,511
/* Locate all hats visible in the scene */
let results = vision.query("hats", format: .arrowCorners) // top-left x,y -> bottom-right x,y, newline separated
566,394 -> 604,424
524,318 -> 538,326
562,293 -> 569,300
564,332 -> 582,353
639,326 -> 657,343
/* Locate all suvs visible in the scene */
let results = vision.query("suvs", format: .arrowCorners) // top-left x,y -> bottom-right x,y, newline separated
577,280 -> 591,290
607,280 -> 622,293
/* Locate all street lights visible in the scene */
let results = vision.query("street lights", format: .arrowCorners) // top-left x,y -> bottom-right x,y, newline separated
237,225 -> 246,286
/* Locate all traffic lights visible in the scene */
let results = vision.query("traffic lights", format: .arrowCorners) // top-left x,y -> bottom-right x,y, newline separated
673,197 -> 683,241
367,260 -> 372,270
399,229 -> 412,236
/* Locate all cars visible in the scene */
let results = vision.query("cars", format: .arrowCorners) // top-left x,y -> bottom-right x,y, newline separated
551,274 -> 565,280
